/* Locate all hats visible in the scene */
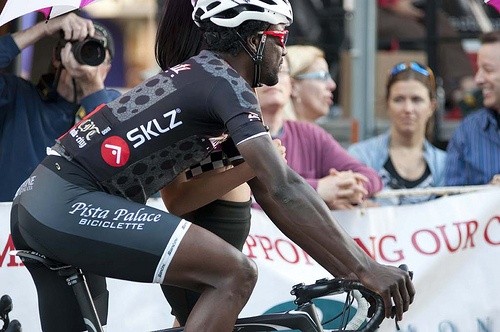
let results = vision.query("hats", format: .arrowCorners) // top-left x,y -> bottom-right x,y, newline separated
55,22 -> 116,62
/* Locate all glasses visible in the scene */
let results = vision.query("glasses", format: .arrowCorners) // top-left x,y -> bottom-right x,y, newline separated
389,60 -> 430,78
257,30 -> 289,47
293,69 -> 332,81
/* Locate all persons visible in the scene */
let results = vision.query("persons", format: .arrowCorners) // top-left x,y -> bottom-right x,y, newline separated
450,74 -> 484,117
347,60 -> 448,208
253,54 -> 382,208
0,12 -> 122,201
444,28 -> 500,195
285,46 -> 336,121
10,0 -> 415,332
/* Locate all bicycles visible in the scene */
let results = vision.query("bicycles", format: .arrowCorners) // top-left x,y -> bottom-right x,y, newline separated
0,250 -> 414,332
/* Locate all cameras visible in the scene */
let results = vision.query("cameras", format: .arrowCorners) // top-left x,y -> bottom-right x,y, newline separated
55,30 -> 106,66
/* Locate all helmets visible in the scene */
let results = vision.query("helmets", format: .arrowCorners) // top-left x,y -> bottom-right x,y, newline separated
189,0 -> 294,29
